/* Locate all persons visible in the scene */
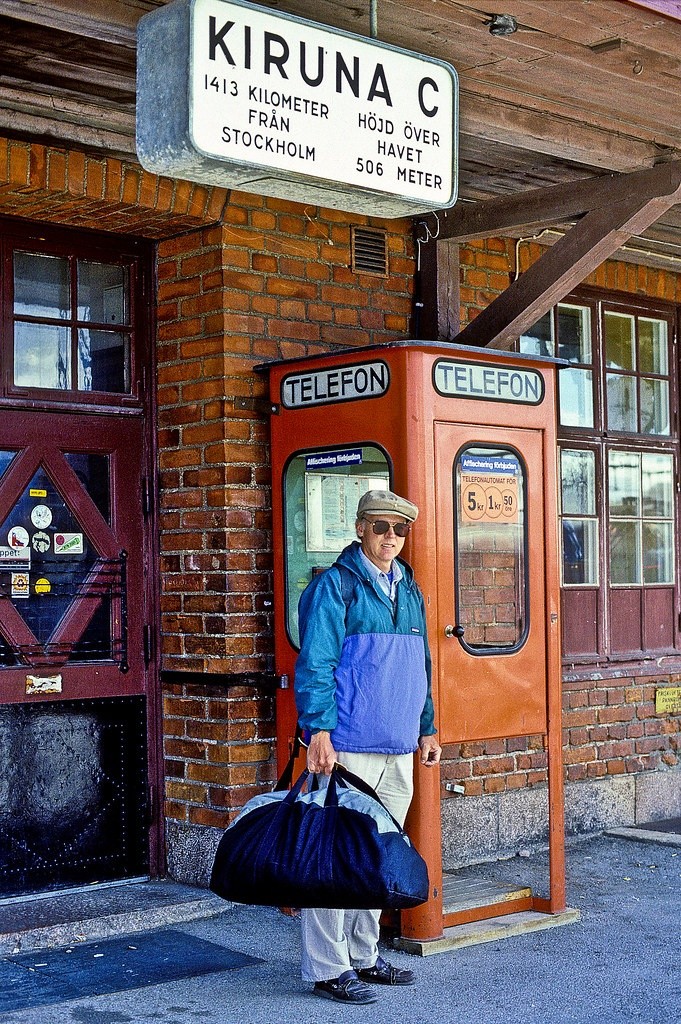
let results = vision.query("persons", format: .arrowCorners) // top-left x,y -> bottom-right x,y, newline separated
294,490 -> 444,1004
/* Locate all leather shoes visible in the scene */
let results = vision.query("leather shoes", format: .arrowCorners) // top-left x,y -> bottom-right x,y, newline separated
353,956 -> 415,986
313,970 -> 378,1004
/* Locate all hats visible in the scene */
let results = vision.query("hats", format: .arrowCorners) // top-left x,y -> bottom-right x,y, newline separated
358,490 -> 418,524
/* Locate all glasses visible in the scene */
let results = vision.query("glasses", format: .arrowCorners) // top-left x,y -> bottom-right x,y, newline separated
360,517 -> 412,537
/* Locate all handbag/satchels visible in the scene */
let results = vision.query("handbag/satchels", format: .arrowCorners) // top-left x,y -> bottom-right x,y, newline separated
209,766 -> 429,909
